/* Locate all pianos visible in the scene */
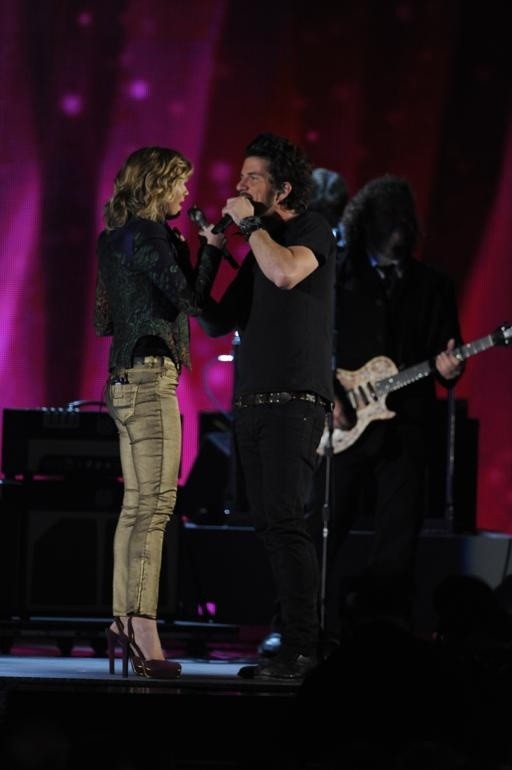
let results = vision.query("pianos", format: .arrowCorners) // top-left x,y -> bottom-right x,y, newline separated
1,410 -> 230,475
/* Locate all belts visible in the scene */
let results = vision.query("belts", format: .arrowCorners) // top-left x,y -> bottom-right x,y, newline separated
234,392 -> 328,408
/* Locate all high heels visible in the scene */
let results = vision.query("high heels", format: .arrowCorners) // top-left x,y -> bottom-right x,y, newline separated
107,611 -> 182,678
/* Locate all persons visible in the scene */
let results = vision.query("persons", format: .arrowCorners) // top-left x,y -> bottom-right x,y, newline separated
171,134 -> 338,680
308,176 -> 466,661
92,146 -> 228,680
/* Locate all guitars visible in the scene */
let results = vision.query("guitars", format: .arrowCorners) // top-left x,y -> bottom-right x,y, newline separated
315,325 -> 511,455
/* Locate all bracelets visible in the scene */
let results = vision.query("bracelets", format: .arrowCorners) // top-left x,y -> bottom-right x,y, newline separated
239,214 -> 263,239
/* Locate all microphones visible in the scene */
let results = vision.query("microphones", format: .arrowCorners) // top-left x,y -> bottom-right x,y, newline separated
211,192 -> 254,236
188,203 -> 240,271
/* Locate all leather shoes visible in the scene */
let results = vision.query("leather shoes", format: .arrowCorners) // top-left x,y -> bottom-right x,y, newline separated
237,655 -> 333,681
